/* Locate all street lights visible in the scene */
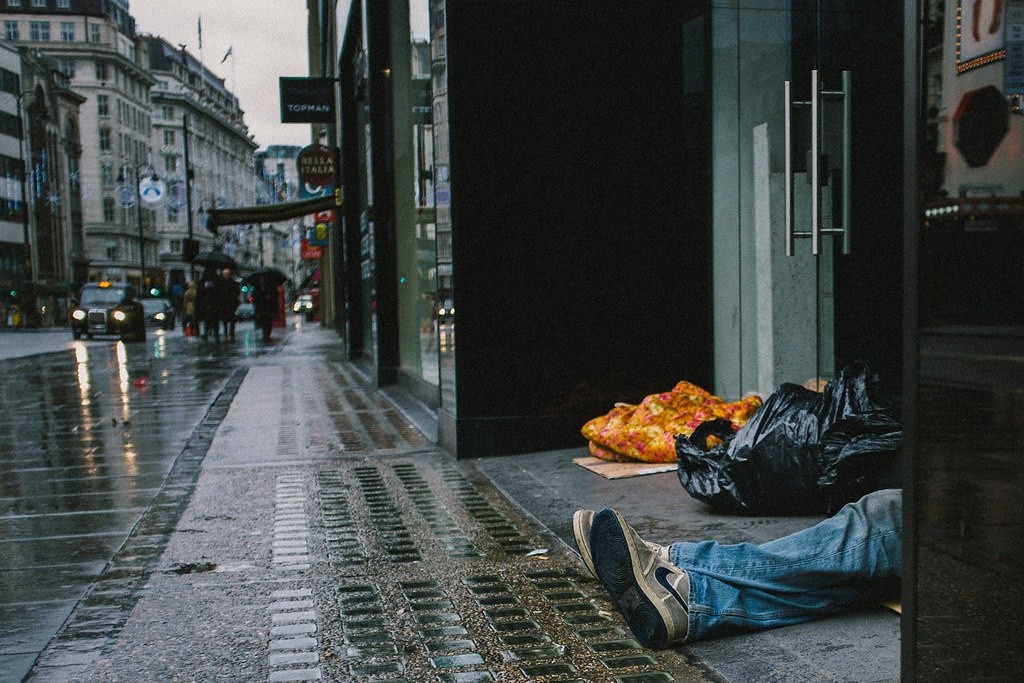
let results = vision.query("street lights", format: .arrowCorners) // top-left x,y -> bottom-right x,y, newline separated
17,82 -> 45,328
196,191 -> 225,252
116,154 -> 160,293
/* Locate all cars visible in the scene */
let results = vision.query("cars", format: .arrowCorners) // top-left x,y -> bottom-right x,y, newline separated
293,294 -> 313,316
235,303 -> 257,323
132,299 -> 177,331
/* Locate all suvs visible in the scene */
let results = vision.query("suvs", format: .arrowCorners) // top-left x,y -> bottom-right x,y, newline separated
70,278 -> 145,340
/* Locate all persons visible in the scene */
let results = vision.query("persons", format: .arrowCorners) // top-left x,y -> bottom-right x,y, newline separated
571,488 -> 903,651
182,267 -> 279,344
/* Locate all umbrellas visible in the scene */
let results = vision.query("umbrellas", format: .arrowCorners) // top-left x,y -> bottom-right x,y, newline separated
242,267 -> 287,287
191,252 -> 236,269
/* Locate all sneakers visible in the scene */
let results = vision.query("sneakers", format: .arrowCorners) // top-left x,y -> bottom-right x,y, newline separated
572,507 -> 691,651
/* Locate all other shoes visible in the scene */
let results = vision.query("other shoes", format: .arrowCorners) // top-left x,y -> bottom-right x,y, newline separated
200,333 -> 210,345
215,336 -> 223,345
230,336 -> 235,343
224,336 -> 229,343
